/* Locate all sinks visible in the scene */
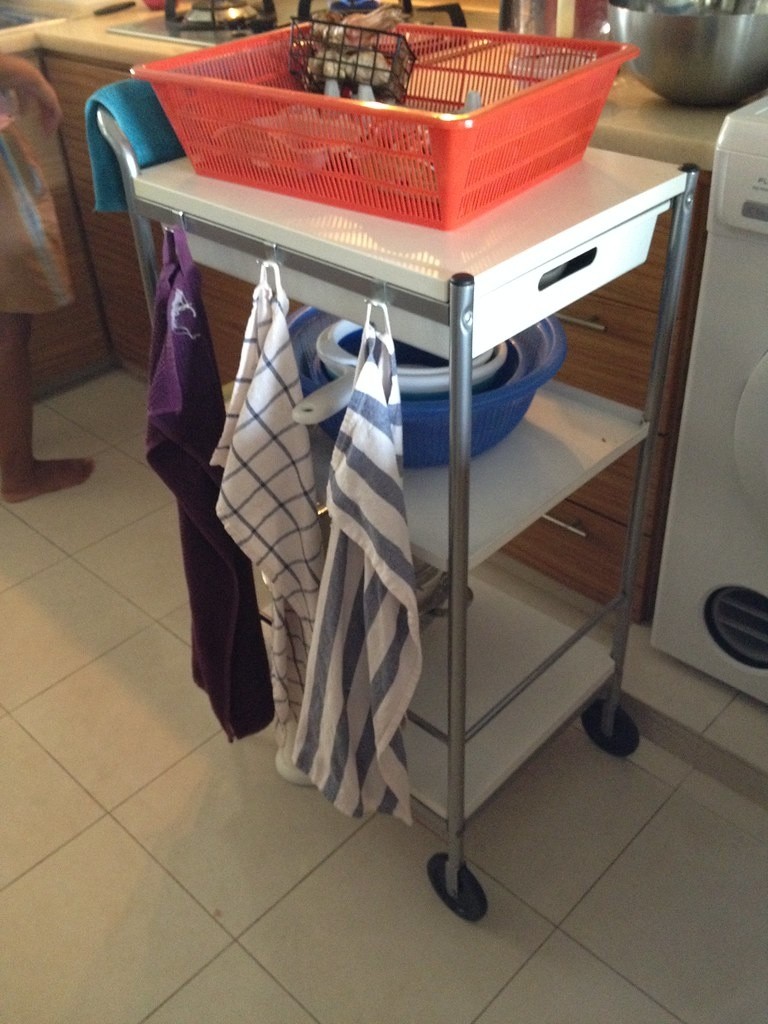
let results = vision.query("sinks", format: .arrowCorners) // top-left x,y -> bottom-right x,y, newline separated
0,10 -> 28,33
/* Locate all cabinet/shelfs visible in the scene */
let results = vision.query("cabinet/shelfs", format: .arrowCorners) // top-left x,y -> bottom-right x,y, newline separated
43,48 -> 255,391
88,103 -> 701,920
501,169 -> 716,624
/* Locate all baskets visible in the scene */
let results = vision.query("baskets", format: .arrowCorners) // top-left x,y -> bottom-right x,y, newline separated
287,305 -> 567,468
129,18 -> 638,230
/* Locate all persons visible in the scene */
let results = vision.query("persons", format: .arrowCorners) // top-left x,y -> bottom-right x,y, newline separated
0,53 -> 95,503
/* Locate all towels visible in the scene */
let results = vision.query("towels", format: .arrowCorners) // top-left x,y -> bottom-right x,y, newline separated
294,301 -> 423,829
82,77 -> 187,215
209,256 -> 322,788
143,217 -> 275,744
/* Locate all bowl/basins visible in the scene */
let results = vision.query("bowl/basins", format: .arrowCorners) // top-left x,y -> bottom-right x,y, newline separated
608,0 -> 768,106
